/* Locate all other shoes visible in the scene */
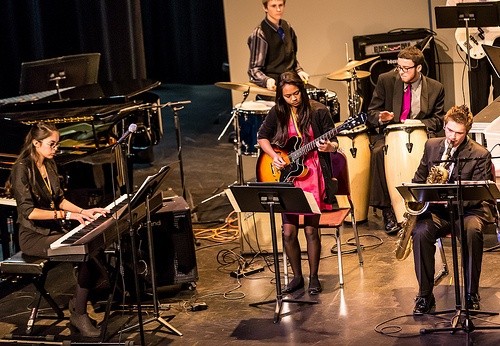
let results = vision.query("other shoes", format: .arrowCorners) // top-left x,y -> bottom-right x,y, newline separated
70,308 -> 101,337
69,298 -> 96,327
281,278 -> 304,293
308,280 -> 322,294
383,208 -> 400,234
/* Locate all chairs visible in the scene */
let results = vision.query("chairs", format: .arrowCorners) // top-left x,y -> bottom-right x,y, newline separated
292,150 -> 363,287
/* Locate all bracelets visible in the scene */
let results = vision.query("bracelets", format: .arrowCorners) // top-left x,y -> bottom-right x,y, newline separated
80,210 -> 83,214
66,211 -> 71,220
54,211 -> 57,219
60,210 -> 67,218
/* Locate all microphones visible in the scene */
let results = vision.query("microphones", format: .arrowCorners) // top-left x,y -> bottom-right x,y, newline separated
111,124 -> 137,149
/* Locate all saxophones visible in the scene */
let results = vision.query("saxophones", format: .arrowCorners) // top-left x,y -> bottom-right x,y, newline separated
395,140 -> 454,261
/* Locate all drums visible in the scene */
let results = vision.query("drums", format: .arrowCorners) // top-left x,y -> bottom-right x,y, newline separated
382,123 -> 428,223
334,121 -> 371,221
234,100 -> 277,156
307,89 -> 339,116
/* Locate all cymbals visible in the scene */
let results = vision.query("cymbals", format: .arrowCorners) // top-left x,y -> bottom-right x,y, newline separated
326,70 -> 372,80
215,82 -> 276,97
329,56 -> 380,73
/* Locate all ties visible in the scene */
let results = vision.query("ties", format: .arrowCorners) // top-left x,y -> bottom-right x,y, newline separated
277,28 -> 285,41
399,84 -> 412,124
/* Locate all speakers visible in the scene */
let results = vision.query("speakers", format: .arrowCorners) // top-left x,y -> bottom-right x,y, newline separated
118,196 -> 199,296
238,211 -> 307,254
352,29 -> 437,113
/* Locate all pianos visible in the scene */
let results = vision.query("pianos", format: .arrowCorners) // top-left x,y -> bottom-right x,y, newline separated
47,190 -> 171,346
0,76 -> 162,219
468,44 -> 500,178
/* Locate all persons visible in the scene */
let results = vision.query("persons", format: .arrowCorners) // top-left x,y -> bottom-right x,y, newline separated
366,47 -> 445,233
410,106 -> 494,315
12,122 -> 107,338
446,0 -> 500,116
257,73 -> 340,295
246,0 -> 309,89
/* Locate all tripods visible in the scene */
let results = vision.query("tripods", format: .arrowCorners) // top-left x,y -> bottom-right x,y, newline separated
117,167 -> 184,337
231,185 -> 318,323
407,156 -> 500,346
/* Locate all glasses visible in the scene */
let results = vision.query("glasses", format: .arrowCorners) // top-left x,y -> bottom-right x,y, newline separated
39,140 -> 61,148
396,65 -> 417,73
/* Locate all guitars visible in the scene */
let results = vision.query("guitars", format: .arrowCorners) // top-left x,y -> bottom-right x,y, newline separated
255,111 -> 368,183
455,25 -> 500,59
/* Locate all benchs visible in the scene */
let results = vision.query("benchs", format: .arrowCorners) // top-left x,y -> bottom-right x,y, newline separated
0,251 -> 65,335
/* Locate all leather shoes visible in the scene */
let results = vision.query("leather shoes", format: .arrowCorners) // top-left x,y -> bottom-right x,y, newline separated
465,293 -> 480,315
413,293 -> 435,314
